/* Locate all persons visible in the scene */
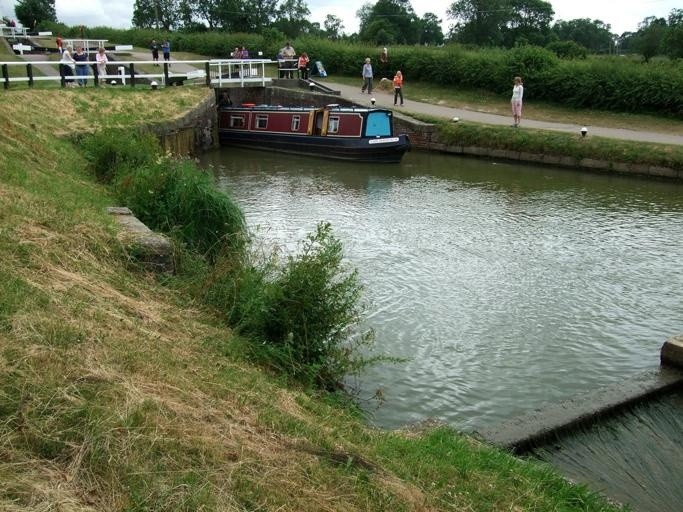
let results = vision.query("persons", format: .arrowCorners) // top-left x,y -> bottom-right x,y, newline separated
511,77 -> 524,127
11,19 -> 15,27
216,92 -> 233,108
62,46 -> 76,87
393,71 -> 405,106
231,43 -> 309,79
74,46 -> 89,88
161,39 -> 171,66
96,48 -> 108,88
362,58 -> 373,94
150,40 -> 160,66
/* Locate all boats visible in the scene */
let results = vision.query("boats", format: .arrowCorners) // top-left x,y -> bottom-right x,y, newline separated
218,104 -> 413,164
